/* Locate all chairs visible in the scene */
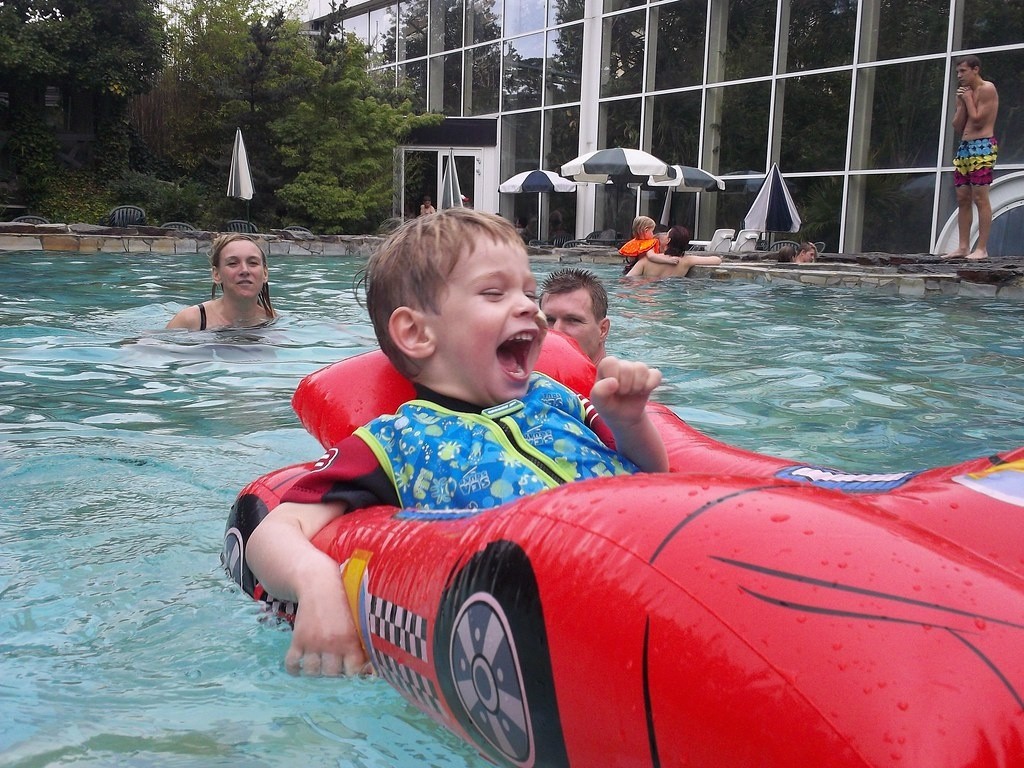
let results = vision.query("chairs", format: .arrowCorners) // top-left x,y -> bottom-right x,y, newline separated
4,200 -> 831,267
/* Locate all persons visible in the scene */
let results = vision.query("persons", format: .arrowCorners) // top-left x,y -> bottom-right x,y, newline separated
243,208 -> 670,677
630,215 -> 680,265
777,246 -> 797,265
165,231 -> 281,332
940,55 -> 999,259
419,195 -> 436,218
538,269 -> 610,368
796,241 -> 818,264
519,209 -> 563,244
624,224 -> 722,277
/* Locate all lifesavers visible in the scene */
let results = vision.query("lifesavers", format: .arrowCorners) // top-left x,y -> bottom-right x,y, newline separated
220,321 -> 1024,768
